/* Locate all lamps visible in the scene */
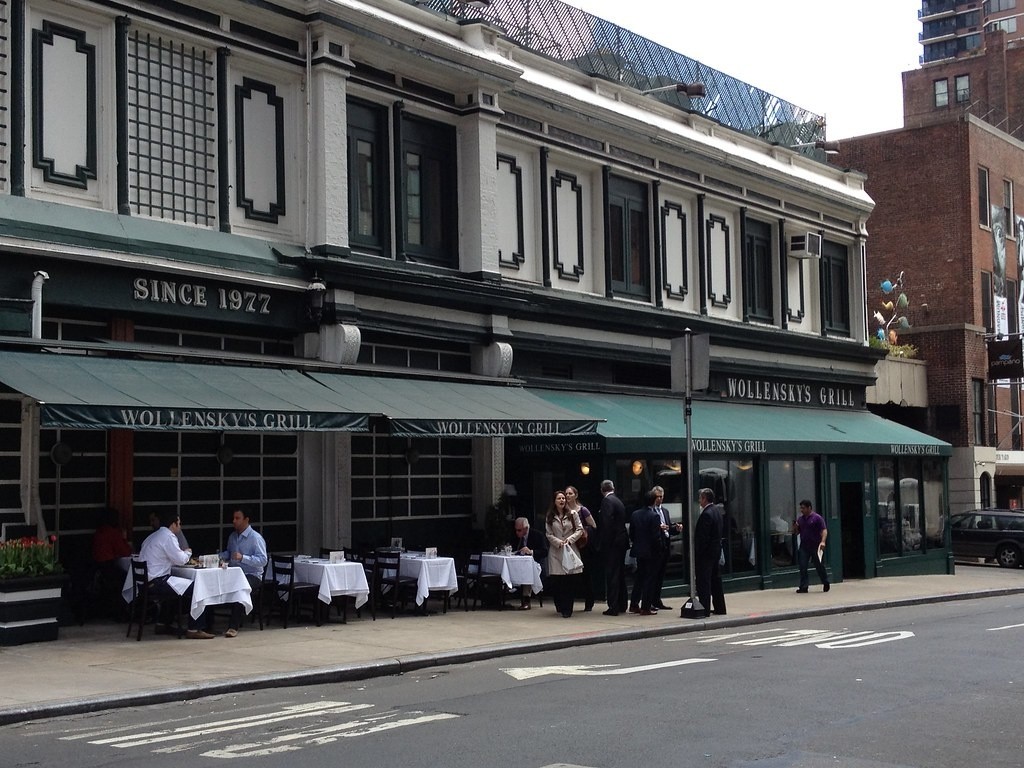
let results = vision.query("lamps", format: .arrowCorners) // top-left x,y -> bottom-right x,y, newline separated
304,270 -> 327,334
790,139 -> 841,154
642,82 -> 706,100
414,0 -> 489,8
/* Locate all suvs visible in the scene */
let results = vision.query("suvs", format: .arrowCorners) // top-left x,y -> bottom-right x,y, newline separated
624,468 -> 753,572
911,508 -> 1024,568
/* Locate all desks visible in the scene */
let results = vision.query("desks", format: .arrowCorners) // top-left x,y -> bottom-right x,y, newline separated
365,551 -> 458,616
116,557 -> 253,634
468,554 -> 543,609
265,557 -> 370,624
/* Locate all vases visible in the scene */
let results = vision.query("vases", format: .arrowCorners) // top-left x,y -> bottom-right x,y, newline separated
0,576 -> 68,646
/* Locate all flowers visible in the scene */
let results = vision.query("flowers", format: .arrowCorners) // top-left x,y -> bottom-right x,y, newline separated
0,534 -> 68,579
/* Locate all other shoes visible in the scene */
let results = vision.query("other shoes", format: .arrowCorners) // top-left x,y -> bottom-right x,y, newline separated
618,607 -> 627,613
824,582 -> 829,592
710,610 -> 726,614
650,606 -> 659,610
657,605 -> 673,609
602,608 -> 618,616
224,628 -> 239,637
519,602 -> 531,610
562,610 -> 572,617
584,607 -> 592,611
797,588 -> 808,593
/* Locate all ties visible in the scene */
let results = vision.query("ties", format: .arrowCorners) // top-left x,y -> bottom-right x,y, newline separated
657,508 -> 669,537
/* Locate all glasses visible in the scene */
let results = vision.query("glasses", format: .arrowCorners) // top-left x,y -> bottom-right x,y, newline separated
800,507 -> 806,509
514,527 -> 522,532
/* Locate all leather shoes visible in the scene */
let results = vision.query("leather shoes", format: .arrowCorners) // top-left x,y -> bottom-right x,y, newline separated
640,609 -> 657,615
629,604 -> 641,612
155,623 -> 179,635
186,629 -> 216,638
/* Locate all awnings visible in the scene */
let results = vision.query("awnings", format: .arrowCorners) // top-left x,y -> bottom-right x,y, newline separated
0,349 -> 606,438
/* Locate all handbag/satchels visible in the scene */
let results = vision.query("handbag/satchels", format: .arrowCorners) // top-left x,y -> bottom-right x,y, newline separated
568,512 -> 588,549
561,543 -> 583,574
718,548 -> 725,565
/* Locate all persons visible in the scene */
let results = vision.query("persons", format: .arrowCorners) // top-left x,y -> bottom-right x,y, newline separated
792,500 -> 829,593
506,479 -> 683,618
197,508 -> 268,637
694,488 -> 726,616
93,506 -> 216,639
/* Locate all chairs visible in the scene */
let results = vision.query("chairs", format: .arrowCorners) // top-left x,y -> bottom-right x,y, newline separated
240,547 -> 543,631
127,558 -> 182,641
